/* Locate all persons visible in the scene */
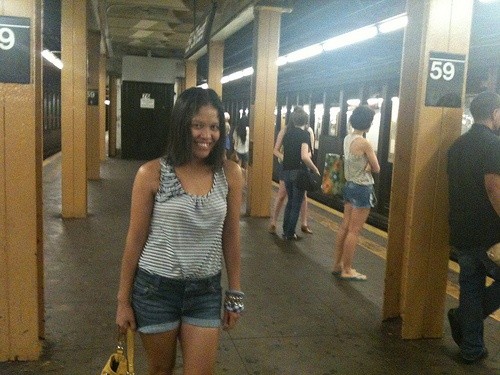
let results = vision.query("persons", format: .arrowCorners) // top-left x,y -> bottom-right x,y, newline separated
232,115 -> 250,186
115,86 -> 245,375
281,109 -> 321,241
447,92 -> 500,367
333,105 -> 379,282
223,111 -> 230,160
270,107 -> 315,234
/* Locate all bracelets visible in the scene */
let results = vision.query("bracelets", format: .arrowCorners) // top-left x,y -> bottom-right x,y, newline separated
224,289 -> 245,314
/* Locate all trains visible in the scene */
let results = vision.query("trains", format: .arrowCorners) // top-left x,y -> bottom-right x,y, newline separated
222,0 -> 500,264
43,56 -> 62,160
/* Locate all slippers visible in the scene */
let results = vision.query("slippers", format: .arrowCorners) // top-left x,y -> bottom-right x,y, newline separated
332,271 -> 343,276
338,273 -> 367,282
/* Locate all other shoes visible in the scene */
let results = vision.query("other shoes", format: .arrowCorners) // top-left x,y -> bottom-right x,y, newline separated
301,225 -> 312,234
269,224 -> 275,234
286,233 -> 302,240
448,307 -> 464,348
461,348 -> 488,362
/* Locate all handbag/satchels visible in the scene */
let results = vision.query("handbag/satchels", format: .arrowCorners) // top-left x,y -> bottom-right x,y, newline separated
99,328 -> 136,375
321,152 -> 346,196
230,151 -> 239,162
308,173 -> 322,192
